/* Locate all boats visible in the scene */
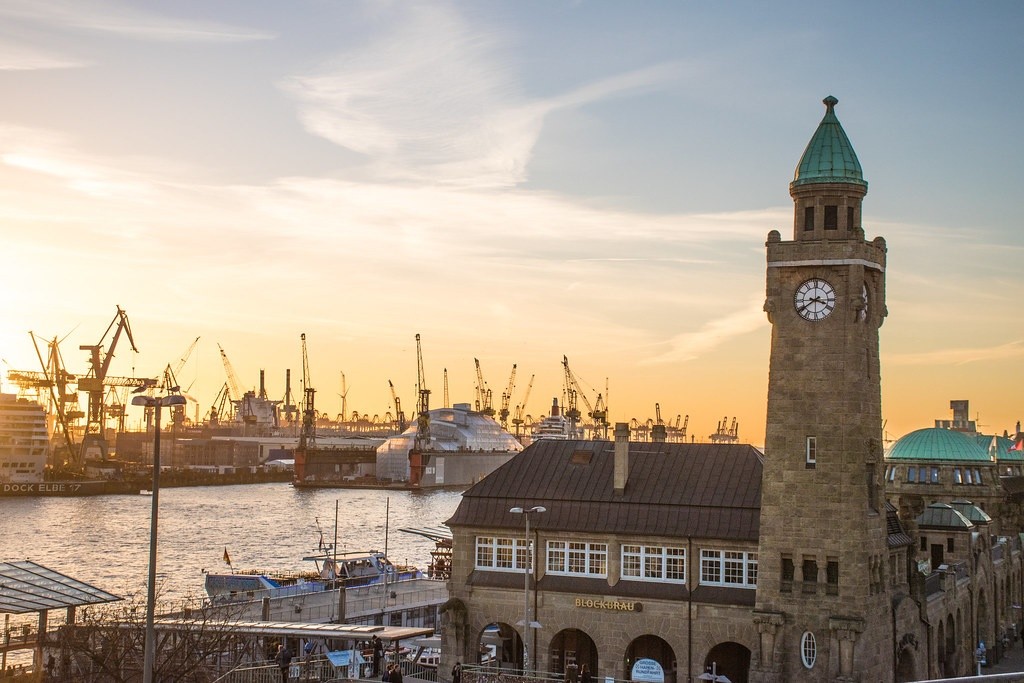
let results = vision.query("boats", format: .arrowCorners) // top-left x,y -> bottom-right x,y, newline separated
405,650 -> 442,667
531,397 -> 574,446
361,646 -> 413,660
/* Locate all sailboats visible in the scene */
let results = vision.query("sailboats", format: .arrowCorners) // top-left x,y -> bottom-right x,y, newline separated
202,500 -> 416,603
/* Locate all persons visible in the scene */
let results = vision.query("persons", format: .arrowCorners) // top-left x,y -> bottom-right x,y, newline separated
385,664 -> 393,681
452,662 -> 461,683
275,644 -> 291,683
564,659 -> 579,683
371,634 -> 382,677
390,663 -> 404,683
581,663 -> 591,683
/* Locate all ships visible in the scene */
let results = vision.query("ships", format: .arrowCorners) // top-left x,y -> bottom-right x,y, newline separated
1,392 -> 142,493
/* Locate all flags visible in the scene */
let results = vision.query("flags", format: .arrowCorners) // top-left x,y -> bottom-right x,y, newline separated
223,547 -> 232,565
1007,439 -> 1023,453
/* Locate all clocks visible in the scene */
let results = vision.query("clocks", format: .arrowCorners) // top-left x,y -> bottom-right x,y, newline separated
862,281 -> 871,322
794,277 -> 837,323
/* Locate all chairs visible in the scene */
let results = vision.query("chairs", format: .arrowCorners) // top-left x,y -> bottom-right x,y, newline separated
235,568 -> 321,587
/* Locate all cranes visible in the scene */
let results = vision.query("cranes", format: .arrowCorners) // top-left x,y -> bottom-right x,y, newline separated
560,361 -> 609,441
443,369 -> 449,408
200,343 -> 259,435
515,374 -> 535,437
412,332 -> 430,452
564,354 -> 580,435
473,357 -> 497,419
301,333 -> 315,434
709,416 -> 740,444
337,371 -> 351,436
6,331 -> 157,457
77,305 -> 140,474
628,413 -> 689,442
147,337 -> 200,433
387,379 -> 406,437
500,364 -> 517,429
